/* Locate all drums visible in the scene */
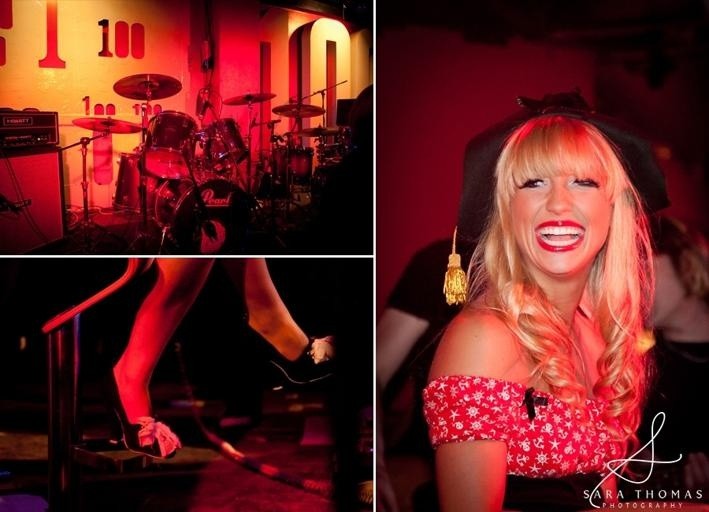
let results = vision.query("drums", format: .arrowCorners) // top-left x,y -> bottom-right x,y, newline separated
270,145 -> 314,187
152,178 -> 252,250
113,152 -> 159,212
134,111 -> 200,180
200,117 -> 248,174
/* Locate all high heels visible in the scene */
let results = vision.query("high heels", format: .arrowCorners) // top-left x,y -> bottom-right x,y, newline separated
109,369 -> 182,459
267,337 -> 342,386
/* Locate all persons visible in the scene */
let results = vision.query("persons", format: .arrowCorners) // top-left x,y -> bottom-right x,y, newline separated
377,89 -> 709,511
104,258 -> 335,460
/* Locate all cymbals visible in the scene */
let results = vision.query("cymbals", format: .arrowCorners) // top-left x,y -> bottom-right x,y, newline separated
114,74 -> 183,100
73,118 -> 142,134
272,103 -> 325,118
222,93 -> 277,106
293,126 -> 340,137
